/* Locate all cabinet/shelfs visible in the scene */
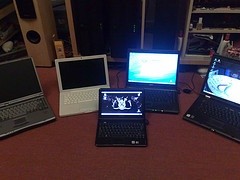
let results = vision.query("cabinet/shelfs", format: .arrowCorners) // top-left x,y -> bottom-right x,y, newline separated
179,0 -> 240,66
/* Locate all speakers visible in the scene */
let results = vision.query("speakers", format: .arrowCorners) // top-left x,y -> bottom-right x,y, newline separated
13,0 -> 59,67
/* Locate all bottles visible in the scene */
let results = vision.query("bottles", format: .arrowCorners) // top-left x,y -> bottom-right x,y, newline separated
218,34 -> 231,57
210,48 -> 215,57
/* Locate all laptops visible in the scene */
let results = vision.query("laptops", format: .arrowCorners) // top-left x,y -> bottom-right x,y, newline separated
55,54 -> 111,117
183,55 -> 240,144
0,57 -> 56,140
124,49 -> 181,113
94,88 -> 148,147
